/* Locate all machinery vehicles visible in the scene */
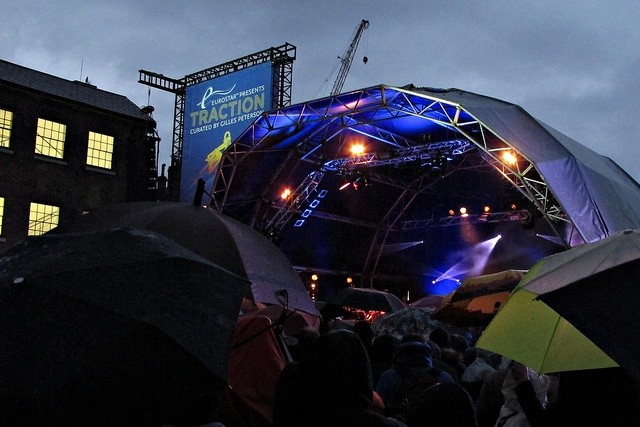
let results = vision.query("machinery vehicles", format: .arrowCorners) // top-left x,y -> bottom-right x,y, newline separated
313,18 -> 370,98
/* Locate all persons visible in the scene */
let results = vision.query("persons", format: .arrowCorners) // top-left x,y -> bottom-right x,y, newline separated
276,316 -> 549,425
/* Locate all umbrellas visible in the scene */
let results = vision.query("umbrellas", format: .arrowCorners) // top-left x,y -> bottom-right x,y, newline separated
448,268 -> 537,312
43,178 -> 326,321
408,293 -> 444,308
329,288 -> 406,314
0,226 -> 251,426
475,229 -> 639,374
